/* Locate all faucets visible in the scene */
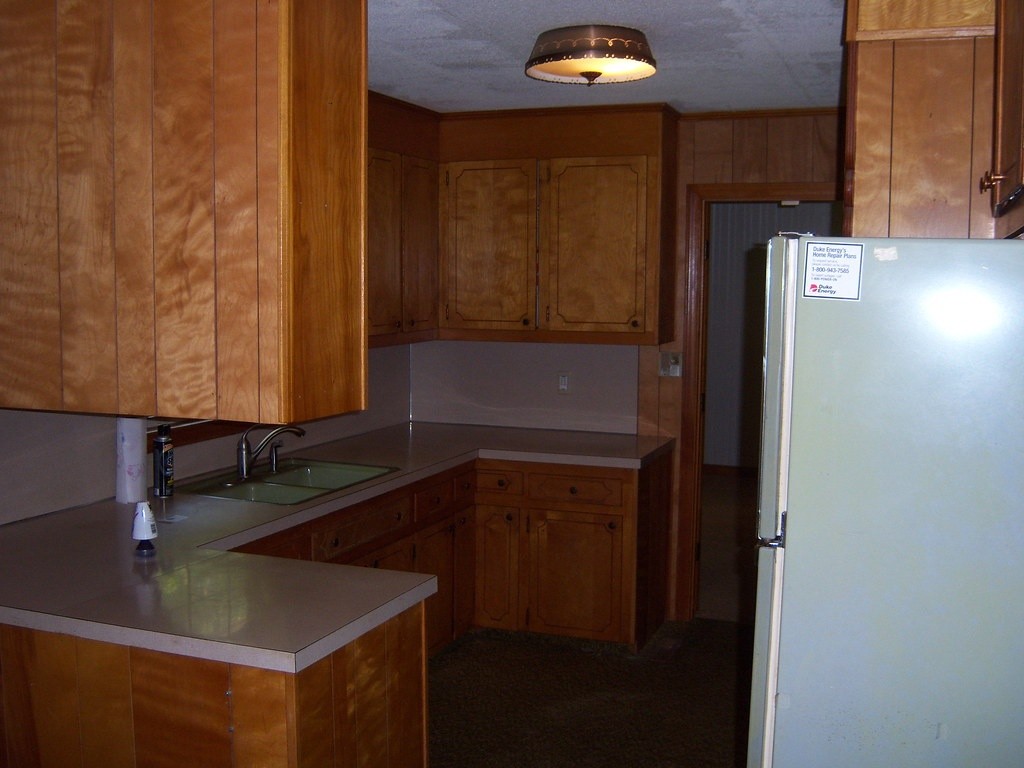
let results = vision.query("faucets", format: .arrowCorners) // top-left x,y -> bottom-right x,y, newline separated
237,423 -> 306,479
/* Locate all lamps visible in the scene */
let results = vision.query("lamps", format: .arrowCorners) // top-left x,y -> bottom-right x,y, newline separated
524,24 -> 656,86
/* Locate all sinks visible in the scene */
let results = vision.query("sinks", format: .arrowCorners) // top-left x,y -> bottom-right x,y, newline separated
261,456 -> 400,490
190,481 -> 332,506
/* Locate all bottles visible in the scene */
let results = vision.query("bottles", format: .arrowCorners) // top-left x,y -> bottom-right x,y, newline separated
152,423 -> 174,500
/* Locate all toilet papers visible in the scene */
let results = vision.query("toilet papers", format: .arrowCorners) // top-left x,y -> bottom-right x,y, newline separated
116,416 -> 149,504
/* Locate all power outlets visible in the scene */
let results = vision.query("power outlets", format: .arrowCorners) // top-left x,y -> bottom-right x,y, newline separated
557,371 -> 573,395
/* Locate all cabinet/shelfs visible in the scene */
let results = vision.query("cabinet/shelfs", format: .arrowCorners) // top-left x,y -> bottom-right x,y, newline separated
0,1 -> 368,425
476,450 -> 673,653
1,598 -> 433,768
439,102 -> 680,346
367,90 -> 440,348
228,458 -> 477,661
843,1 -> 1023,240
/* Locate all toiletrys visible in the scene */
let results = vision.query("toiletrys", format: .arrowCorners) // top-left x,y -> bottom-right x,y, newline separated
152,424 -> 174,498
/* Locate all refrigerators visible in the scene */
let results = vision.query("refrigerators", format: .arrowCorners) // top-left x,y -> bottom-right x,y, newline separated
747,228 -> 1024,768
